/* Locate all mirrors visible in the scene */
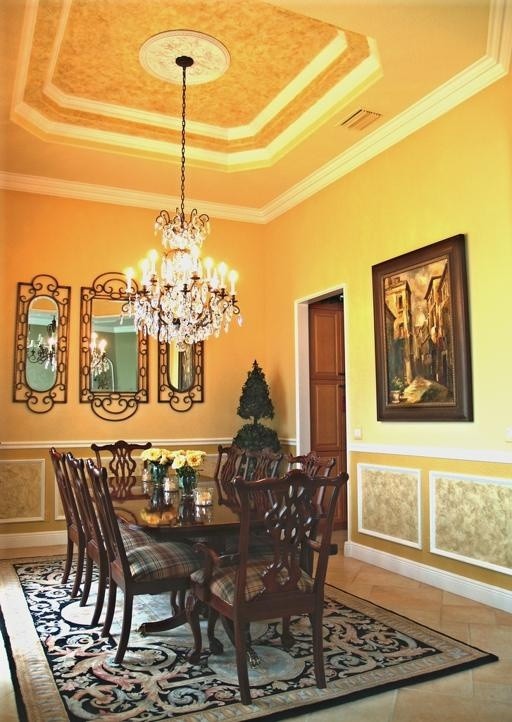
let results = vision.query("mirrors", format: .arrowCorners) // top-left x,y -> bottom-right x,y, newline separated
10,273 -> 72,413
156,294 -> 207,409
79,270 -> 151,421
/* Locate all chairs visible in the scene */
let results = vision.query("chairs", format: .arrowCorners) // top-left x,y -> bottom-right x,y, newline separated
49,441 -> 350,665
185,469 -> 349,703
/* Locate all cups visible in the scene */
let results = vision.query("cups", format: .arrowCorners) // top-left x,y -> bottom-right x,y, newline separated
141,468 -> 154,483
191,486 -> 215,507
163,477 -> 179,493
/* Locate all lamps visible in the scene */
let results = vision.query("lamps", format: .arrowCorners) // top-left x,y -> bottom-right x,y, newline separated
122,56 -> 243,351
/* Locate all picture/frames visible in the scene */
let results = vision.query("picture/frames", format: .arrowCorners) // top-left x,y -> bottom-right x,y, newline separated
370,234 -> 473,421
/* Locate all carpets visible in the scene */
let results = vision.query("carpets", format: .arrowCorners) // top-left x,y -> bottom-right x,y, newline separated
0,552 -> 496,722
